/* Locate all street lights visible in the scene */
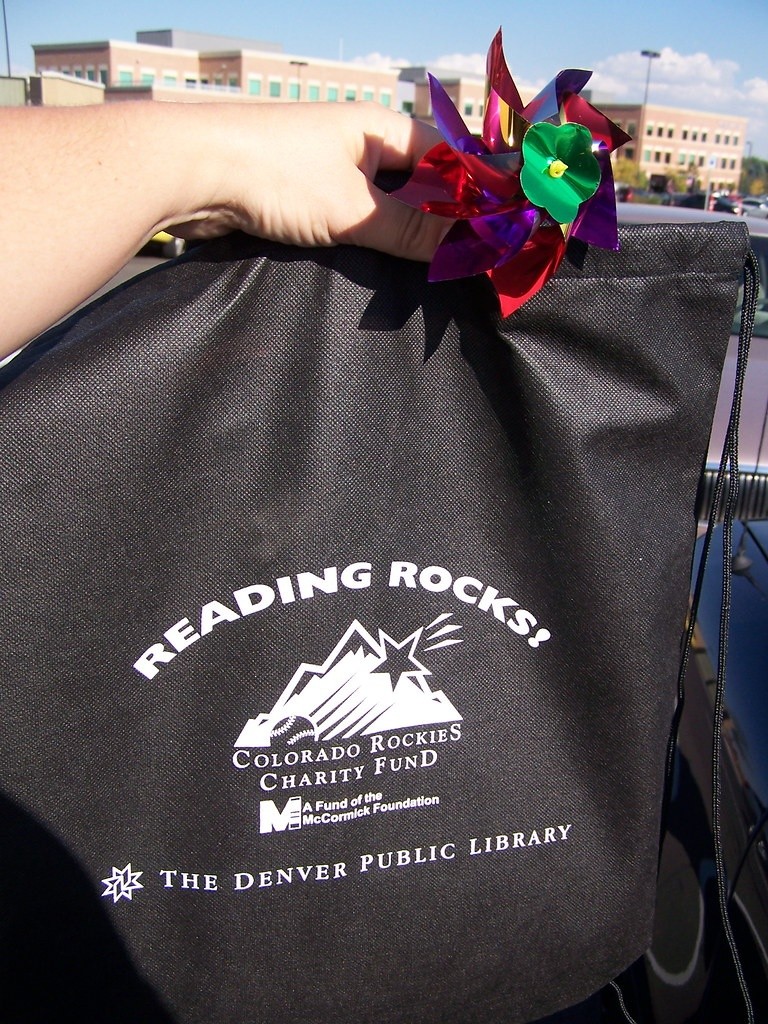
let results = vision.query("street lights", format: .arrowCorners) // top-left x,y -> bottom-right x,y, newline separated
638,50 -> 661,105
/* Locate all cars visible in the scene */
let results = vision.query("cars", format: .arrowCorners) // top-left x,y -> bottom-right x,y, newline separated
646,456 -> 768,1024
612,181 -> 768,469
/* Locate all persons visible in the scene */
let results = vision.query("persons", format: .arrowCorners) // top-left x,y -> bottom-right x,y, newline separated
0,99 -> 512,361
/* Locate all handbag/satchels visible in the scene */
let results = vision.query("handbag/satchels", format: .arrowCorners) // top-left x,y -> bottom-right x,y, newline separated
0,215 -> 761,1024
